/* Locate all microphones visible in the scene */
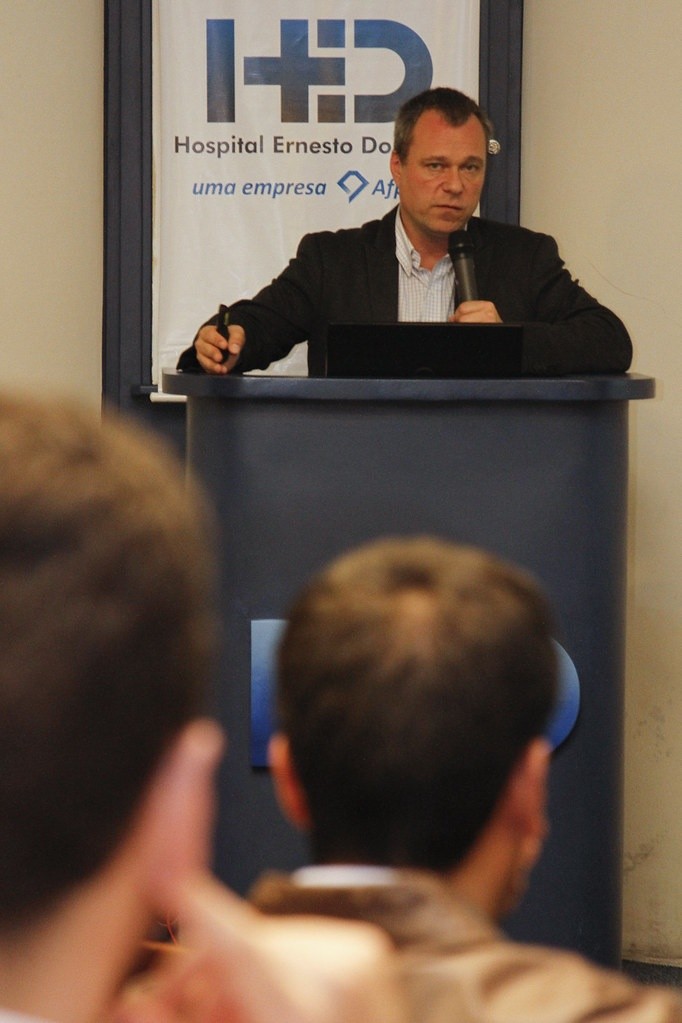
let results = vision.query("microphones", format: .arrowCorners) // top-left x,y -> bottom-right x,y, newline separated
447,229 -> 478,307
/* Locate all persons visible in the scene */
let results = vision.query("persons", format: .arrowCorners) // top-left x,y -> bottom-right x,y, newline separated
167,539 -> 682,1023
0,391 -> 402,1023
176,86 -> 633,377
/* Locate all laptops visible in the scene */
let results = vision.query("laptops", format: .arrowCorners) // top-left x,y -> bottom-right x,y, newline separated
323,319 -> 523,378
